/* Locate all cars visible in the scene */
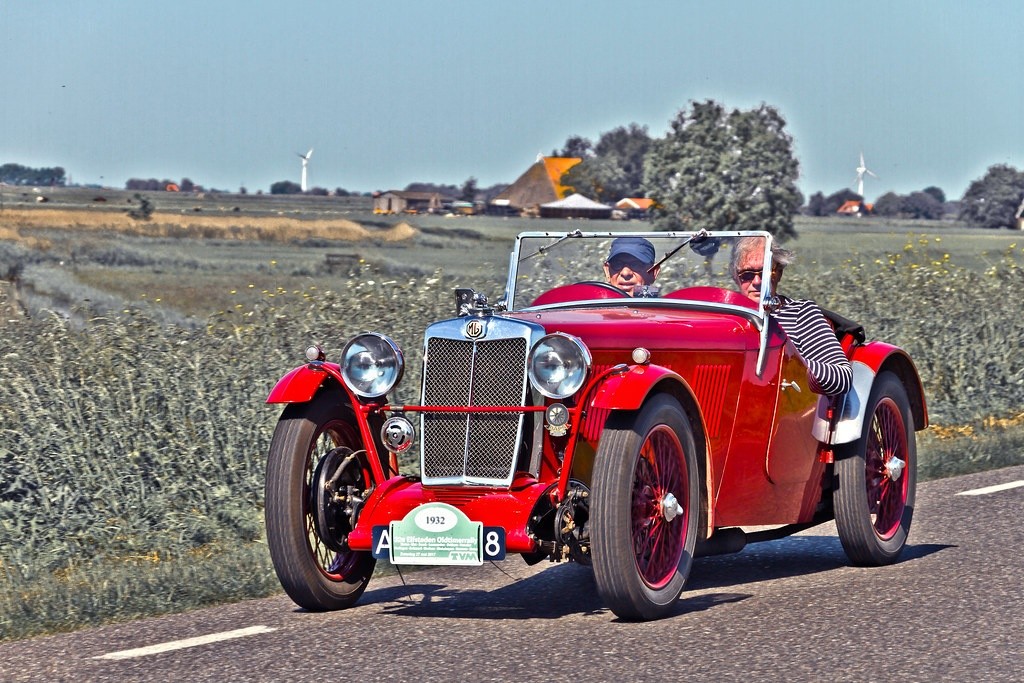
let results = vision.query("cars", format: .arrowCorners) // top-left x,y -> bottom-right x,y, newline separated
263,231 -> 928,619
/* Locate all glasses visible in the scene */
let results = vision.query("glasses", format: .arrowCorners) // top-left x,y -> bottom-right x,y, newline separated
737,266 -> 777,283
610,260 -> 644,272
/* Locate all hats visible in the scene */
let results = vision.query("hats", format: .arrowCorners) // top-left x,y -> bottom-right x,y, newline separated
608,238 -> 655,264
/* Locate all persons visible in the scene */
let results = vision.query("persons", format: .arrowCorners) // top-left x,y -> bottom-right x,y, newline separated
602,237 -> 660,298
730,236 -> 854,394
